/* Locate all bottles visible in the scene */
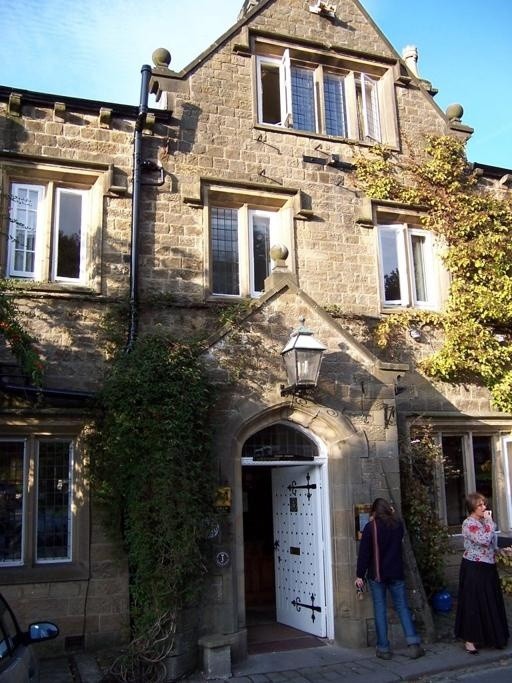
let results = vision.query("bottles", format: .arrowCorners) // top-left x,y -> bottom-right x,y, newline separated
356,584 -> 364,601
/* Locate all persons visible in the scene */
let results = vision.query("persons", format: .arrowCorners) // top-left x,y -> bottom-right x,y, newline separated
355,497 -> 426,661
453,491 -> 510,654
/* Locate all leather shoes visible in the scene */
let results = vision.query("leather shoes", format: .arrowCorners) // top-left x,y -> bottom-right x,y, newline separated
464,642 -> 480,655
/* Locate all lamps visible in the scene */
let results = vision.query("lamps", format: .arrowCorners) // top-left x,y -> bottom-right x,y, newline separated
280,315 -> 327,397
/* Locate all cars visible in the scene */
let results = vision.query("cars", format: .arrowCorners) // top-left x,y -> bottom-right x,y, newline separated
0,593 -> 60,682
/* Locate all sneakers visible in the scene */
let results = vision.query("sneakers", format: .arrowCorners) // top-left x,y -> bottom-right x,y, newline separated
376,649 -> 393,659
408,646 -> 425,658
